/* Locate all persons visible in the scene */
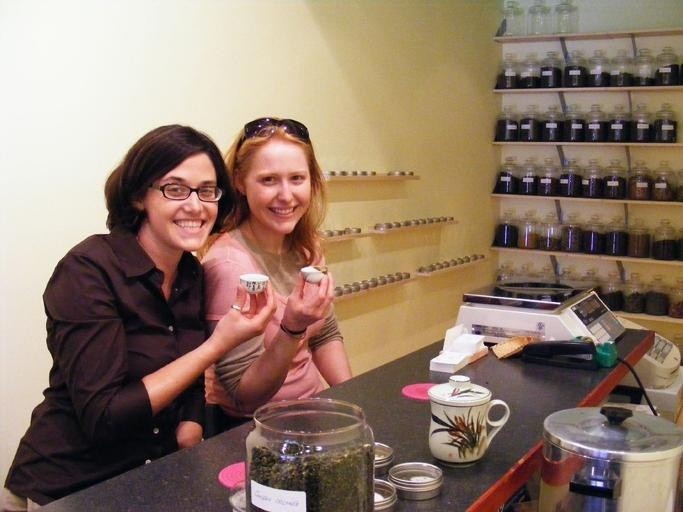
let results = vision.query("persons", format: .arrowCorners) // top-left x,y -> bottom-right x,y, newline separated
0,124 -> 278,512
198,118 -> 352,436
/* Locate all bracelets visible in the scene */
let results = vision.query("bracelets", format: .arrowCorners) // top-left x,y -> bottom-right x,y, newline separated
281,323 -> 307,340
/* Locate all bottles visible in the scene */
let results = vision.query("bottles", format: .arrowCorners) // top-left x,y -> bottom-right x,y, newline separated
244,399 -> 375,511
498,202 -> 679,262
493,150 -> 680,199
496,41 -> 680,91
498,2 -> 585,36
496,266 -> 678,317
497,94 -> 680,145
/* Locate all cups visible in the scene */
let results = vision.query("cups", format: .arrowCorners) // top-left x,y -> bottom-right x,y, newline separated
429,376 -> 509,466
300,266 -> 326,283
239,274 -> 270,293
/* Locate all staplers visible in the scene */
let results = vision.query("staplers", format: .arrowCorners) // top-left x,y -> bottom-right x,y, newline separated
521,340 -> 602,372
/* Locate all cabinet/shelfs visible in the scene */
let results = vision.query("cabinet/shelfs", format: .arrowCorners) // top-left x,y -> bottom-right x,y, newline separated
491,27 -> 683,325
316,176 -> 488,303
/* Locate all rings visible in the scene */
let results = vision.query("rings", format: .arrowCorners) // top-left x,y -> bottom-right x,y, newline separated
230,305 -> 243,313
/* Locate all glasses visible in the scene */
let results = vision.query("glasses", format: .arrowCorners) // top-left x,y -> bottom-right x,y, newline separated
148,183 -> 224,202
233,117 -> 310,164
